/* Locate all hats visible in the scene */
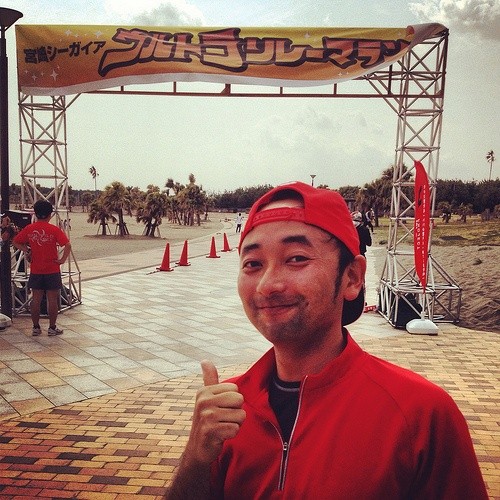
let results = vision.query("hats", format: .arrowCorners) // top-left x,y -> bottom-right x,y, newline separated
350,211 -> 362,222
238,182 -> 365,325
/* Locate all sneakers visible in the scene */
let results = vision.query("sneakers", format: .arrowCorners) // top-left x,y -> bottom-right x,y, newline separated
48,325 -> 63,336
31,325 -> 42,336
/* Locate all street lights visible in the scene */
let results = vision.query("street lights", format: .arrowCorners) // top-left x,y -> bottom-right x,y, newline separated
310,175 -> 317,186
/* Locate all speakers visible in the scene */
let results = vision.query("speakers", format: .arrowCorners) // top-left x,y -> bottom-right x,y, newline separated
8,209 -> 31,240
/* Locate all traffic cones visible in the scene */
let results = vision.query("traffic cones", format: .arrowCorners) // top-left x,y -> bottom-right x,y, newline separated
206,236 -> 221,258
156,242 -> 174,271
221,233 -> 231,252
175,240 -> 191,266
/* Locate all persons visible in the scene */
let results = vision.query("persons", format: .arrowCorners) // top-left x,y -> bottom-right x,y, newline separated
462,207 -> 466,223
0,216 -> 19,249
351,211 -> 371,296
165,182 -> 489,500
441,206 -> 451,224
364,210 -> 373,233
13,201 -> 71,336
235,213 -> 243,233
146,210 -> 162,237
